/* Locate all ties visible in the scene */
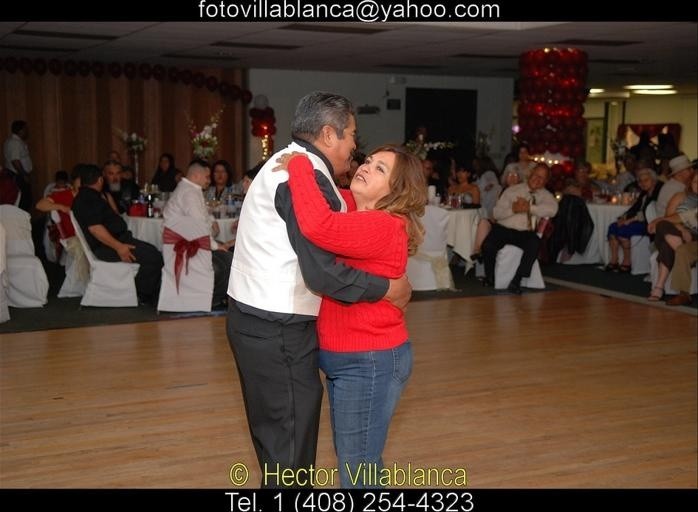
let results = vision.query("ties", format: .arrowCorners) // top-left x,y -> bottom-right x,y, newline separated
527,191 -> 535,231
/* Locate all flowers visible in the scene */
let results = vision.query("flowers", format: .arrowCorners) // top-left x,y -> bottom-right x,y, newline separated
185,103 -> 226,161
108,123 -> 147,151
404,138 -> 458,158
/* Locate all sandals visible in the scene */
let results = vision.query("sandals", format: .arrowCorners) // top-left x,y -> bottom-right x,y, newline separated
648,287 -> 665,301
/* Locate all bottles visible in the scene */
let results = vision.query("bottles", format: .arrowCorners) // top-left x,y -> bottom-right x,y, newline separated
147,195 -> 154,217
139,194 -> 145,204
631,187 -> 636,202
600,188 -> 605,199
606,189 -> 609,201
227,194 -> 236,218
632,192 -> 640,204
435,193 -> 440,206
450,194 -> 459,207
616,190 -> 623,205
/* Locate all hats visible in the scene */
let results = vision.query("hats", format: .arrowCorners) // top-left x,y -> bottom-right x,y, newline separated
667,155 -> 696,176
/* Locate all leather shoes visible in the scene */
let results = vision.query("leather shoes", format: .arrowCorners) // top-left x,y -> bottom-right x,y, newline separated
483,279 -> 491,286
507,282 -> 522,296
666,296 -> 689,305
618,264 -> 631,273
470,253 -> 481,263
606,262 -> 619,271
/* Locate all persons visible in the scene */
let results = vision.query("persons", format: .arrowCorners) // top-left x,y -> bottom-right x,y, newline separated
225,90 -> 356,488
1,126 -> 698,310
270,143 -> 429,489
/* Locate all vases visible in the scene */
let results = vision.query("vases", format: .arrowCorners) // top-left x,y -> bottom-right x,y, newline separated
133,153 -> 141,185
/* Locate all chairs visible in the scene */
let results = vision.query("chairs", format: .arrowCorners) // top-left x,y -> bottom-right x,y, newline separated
14,189 -> 22,208
494,216 -> 545,291
649,250 -> 698,295
70,210 -> 141,311
404,206 -> 452,291
0,204 -> 50,310
44,183 -> 72,263
50,210 -> 90,298
156,215 -> 215,316
0,222 -> 13,324
618,234 -> 652,275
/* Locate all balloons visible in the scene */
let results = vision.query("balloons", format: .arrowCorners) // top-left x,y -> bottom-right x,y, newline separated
2,56 -> 276,138
514,48 -> 587,195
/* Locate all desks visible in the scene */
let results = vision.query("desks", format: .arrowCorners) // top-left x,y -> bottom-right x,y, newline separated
118,200 -> 238,254
426,197 -> 489,276
556,193 -> 643,265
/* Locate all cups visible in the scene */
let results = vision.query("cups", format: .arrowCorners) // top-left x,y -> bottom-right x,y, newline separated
213,207 -> 221,219
457,196 -> 463,208
